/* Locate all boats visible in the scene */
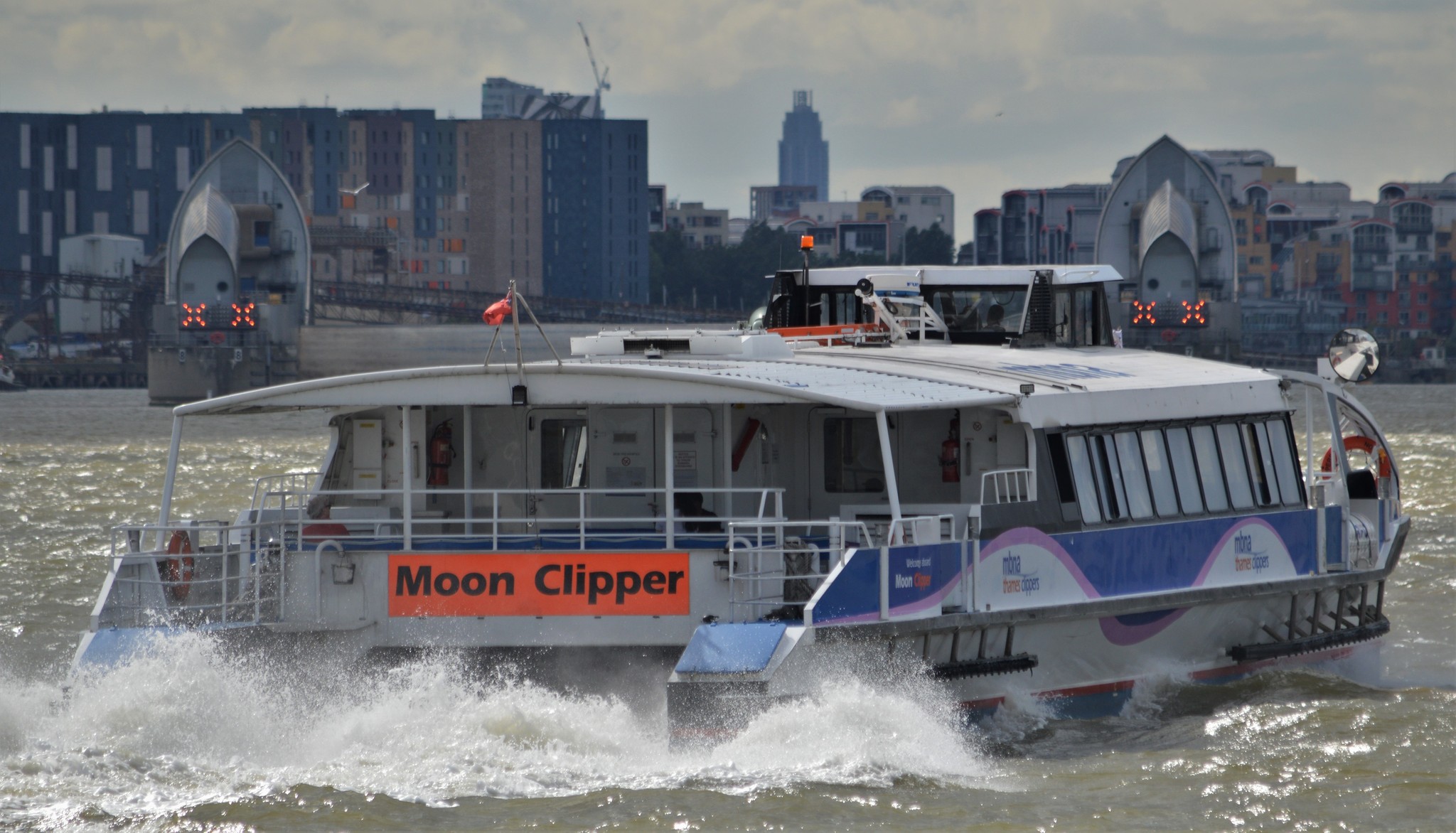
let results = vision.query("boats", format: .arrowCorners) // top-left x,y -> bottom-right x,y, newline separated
64,231 -> 1414,758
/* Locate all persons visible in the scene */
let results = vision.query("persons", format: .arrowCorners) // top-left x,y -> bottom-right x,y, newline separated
951,306 -> 978,331
655,492 -> 721,533
981,305 -> 1006,332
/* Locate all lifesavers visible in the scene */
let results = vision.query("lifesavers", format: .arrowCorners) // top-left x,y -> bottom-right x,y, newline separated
1162,329 -> 1176,342
1320,436 -> 1391,493
168,530 -> 192,601
210,332 -> 224,344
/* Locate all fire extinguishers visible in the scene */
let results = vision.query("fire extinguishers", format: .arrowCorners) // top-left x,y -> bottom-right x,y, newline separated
939,431 -> 958,483
429,417 -> 453,486
